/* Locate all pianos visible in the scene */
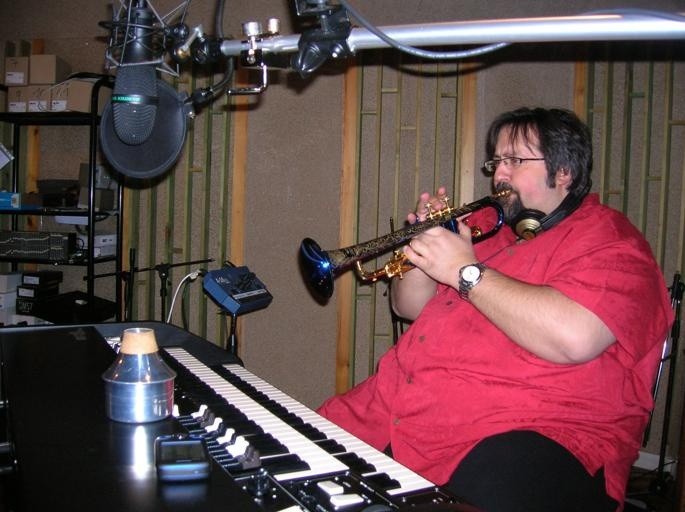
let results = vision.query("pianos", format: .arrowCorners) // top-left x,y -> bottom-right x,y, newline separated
0,321 -> 482,512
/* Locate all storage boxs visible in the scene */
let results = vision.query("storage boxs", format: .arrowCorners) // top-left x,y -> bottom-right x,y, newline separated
0,193 -> 43,210
0,38 -> 111,115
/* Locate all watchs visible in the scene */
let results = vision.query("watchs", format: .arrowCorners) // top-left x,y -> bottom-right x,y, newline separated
456,261 -> 486,300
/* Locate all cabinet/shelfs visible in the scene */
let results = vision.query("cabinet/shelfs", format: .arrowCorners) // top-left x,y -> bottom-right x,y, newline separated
0,78 -> 123,323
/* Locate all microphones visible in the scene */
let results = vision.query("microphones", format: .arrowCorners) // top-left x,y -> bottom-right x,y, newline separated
112,9 -> 161,147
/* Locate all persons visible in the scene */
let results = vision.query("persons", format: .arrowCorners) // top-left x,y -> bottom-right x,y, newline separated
313,107 -> 676,512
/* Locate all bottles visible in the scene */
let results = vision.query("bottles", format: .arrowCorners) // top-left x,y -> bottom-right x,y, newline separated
102,327 -> 177,423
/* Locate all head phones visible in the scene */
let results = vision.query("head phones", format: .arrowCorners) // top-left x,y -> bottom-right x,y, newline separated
512,176 -> 593,241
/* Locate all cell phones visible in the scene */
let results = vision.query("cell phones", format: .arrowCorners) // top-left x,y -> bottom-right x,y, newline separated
155,433 -> 212,484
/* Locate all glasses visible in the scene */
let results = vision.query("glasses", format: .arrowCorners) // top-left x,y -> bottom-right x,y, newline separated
483,156 -> 544,173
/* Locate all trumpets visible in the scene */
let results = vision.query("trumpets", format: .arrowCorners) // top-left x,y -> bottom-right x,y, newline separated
297,190 -> 516,307
